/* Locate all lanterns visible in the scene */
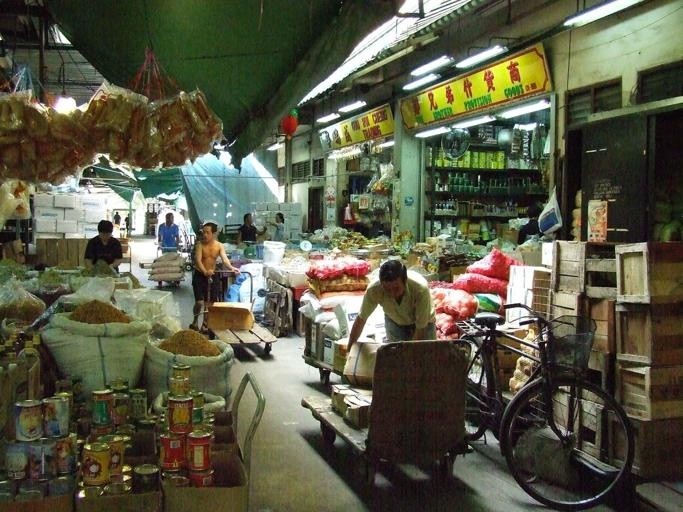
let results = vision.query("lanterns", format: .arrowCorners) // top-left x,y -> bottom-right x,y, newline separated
279,113 -> 297,135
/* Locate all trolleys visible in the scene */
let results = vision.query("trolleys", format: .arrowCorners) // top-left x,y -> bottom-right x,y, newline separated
302,294 -> 394,385
153,246 -> 185,289
206,267 -> 278,359
301,338 -> 472,487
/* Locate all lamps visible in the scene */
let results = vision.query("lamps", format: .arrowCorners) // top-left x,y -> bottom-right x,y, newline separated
455,34 -> 524,70
379,141 -> 393,148
411,55 -> 454,77
316,113 -> 339,124
499,101 -> 551,120
451,116 -> 496,131
338,101 -> 366,113
403,73 -> 439,94
54,62 -> 76,114
561,0 -> 643,29
416,127 -> 451,139
267,144 -> 283,151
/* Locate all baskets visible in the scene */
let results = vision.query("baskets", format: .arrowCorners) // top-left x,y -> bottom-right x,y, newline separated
539,315 -> 597,381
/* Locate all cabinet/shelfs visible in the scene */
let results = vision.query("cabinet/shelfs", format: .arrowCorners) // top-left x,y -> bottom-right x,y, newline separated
426,144 -> 548,244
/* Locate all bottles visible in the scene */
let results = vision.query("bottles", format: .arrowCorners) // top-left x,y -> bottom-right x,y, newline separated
435,200 -> 457,216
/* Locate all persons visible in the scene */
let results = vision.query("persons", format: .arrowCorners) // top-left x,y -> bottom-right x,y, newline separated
189,222 -> 241,332
125,213 -> 129,235
238,212 -> 267,247
347,260 -> 435,353
157,213 -> 180,252
518,201 -> 544,244
85,220 -> 122,274
114,212 -> 121,225
266,213 -> 285,242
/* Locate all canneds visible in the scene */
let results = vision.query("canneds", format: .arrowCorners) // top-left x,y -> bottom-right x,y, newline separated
0,363 -> 215,498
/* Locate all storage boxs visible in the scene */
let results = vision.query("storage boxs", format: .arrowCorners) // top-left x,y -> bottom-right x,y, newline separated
1,354 -> 42,399
255,202 -> 302,241
0,364 -> 28,439
305,301 -> 384,430
26,193 -> 121,257
193,300 -> 254,329
0,410 -> 249,512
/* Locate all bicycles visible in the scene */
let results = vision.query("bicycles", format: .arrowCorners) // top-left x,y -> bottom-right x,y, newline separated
452,300 -> 635,505
